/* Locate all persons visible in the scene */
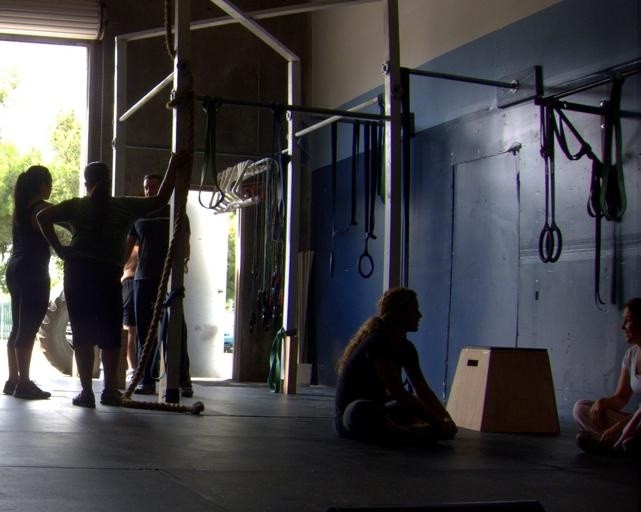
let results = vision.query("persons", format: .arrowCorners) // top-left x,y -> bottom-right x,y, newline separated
2,164 -> 70,400
35,147 -> 194,408
332,284 -> 458,447
119,234 -> 141,383
572,295 -> 641,453
126,169 -> 196,398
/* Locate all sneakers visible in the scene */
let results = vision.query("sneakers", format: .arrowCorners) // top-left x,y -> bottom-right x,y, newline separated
181,390 -> 193,398
4,380 -> 17,395
73,390 -> 95,407
573,429 -> 641,458
134,385 -> 157,395
15,381 -> 51,398
100,388 -> 123,405
